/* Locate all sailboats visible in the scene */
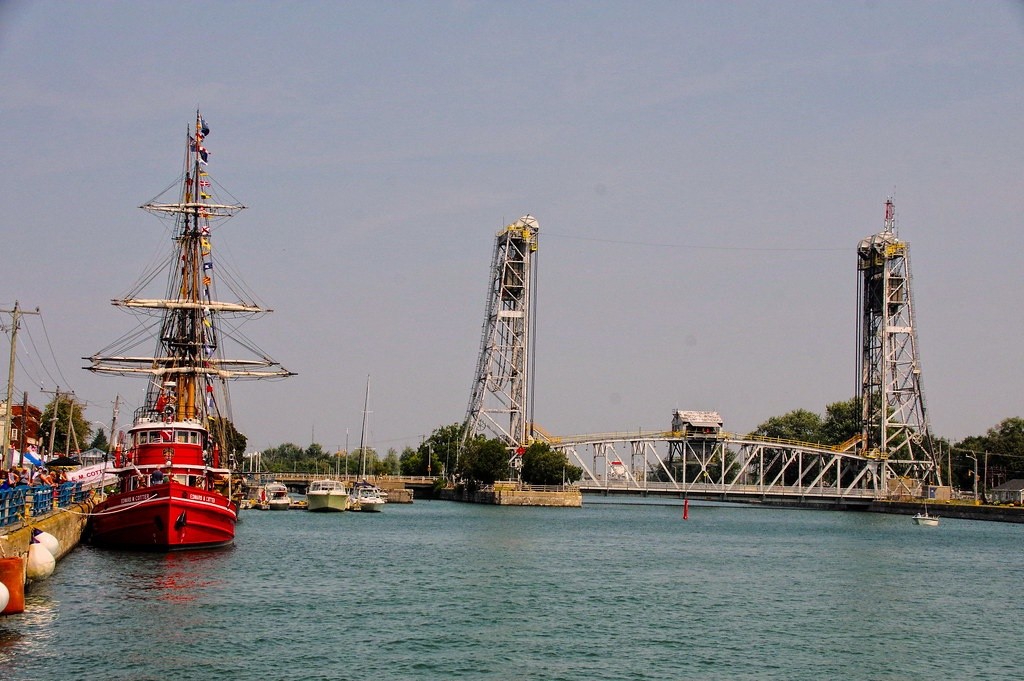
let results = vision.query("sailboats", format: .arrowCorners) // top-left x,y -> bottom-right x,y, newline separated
239,375 -> 390,513
80,102 -> 300,555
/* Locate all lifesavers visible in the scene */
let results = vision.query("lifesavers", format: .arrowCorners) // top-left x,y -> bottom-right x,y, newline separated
115,445 -> 122,469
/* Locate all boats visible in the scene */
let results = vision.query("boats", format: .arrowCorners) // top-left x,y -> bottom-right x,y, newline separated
912,501 -> 939,526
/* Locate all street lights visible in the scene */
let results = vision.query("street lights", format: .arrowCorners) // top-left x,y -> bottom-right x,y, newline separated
93,420 -> 134,461
965,455 -> 977,501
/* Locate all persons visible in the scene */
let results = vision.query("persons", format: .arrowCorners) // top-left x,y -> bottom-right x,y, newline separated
1,466 -> 76,490
118,473 -> 126,495
152,466 -> 165,484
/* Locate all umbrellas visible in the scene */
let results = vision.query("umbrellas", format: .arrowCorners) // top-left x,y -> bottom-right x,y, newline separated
45,455 -> 82,467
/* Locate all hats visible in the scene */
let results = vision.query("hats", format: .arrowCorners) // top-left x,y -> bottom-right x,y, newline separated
43,468 -> 47,471
50,470 -> 56,476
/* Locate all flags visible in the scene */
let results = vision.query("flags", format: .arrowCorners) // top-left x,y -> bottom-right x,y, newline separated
190,109 -> 213,286
